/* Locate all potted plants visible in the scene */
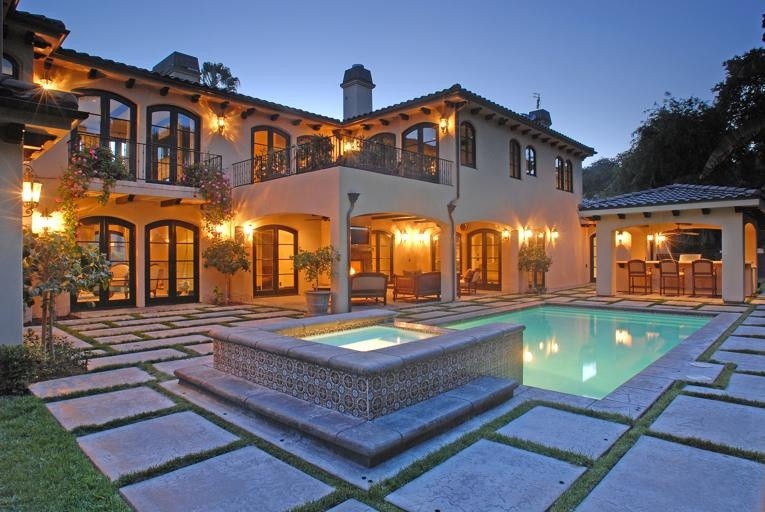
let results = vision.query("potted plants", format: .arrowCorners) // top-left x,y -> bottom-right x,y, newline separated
288,243 -> 341,314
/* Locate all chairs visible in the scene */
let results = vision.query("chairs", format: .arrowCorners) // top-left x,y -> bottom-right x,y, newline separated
457,268 -> 482,299
617,253 -> 753,297
105,260 -> 160,300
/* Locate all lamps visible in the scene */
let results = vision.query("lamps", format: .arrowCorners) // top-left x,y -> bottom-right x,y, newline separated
440,113 -> 448,134
22,163 -> 44,217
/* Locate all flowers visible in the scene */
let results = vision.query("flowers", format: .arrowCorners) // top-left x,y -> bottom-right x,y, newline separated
180,161 -> 236,231
52,143 -> 135,239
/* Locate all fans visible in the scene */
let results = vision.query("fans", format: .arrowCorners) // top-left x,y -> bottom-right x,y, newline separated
659,223 -> 700,237
304,215 -> 330,222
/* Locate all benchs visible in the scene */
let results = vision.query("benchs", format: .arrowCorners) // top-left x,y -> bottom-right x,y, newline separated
351,272 -> 389,306
393,269 -> 441,304
